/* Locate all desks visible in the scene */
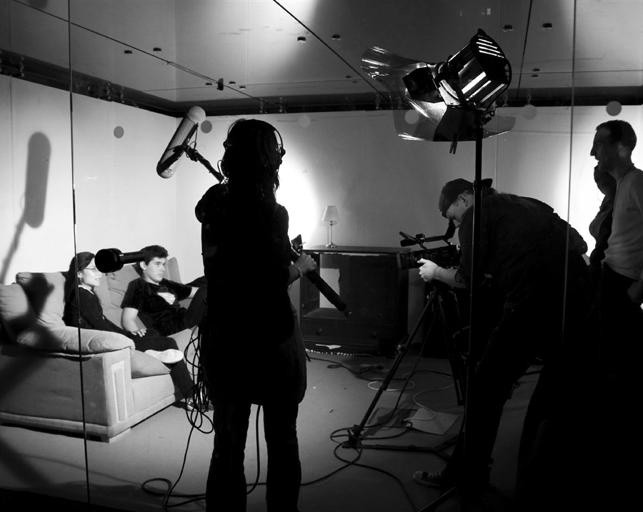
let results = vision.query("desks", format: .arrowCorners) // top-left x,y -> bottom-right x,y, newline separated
287,245 -> 428,357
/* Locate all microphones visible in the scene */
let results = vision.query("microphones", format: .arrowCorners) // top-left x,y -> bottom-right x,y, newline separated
93,249 -> 149,275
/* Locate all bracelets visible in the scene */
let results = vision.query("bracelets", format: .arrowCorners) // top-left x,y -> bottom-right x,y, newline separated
295,263 -> 305,279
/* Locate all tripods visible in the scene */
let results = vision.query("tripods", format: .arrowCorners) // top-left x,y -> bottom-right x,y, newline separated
356,281 -> 465,433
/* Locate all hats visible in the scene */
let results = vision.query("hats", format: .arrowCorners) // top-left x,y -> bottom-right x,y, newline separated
439,178 -> 494,218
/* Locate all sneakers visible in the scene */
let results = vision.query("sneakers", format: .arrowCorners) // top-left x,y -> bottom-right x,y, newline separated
144,349 -> 184,364
413,471 -> 443,486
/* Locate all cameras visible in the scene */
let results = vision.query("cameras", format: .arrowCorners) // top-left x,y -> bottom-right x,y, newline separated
396,216 -> 462,292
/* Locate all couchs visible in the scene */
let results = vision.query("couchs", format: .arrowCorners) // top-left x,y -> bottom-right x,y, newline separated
0,257 -> 200,443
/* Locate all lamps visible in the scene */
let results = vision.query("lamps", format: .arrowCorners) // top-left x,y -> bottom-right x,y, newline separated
361,26 -> 515,511
321,205 -> 339,249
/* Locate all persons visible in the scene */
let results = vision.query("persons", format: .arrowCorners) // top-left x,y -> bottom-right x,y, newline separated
63,252 -> 212,412
119,246 -> 207,388
409,120 -> 643,509
195,119 -> 318,510
